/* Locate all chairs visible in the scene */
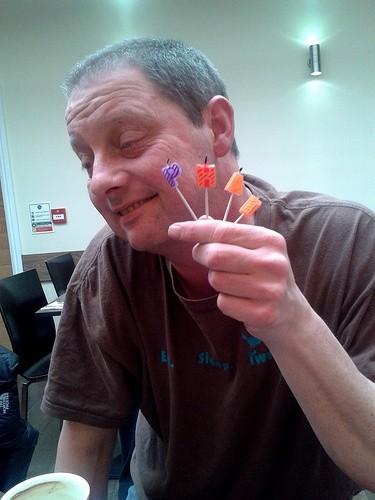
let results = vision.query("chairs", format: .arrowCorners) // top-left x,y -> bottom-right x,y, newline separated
1,269 -> 64,432
45,252 -> 76,297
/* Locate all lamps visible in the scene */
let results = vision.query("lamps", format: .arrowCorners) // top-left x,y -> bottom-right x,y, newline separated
307,44 -> 322,76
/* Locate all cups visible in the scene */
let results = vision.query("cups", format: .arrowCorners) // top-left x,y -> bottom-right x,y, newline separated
0,471 -> 91,500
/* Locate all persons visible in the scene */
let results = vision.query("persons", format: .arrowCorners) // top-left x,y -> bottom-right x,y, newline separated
38,36 -> 375,500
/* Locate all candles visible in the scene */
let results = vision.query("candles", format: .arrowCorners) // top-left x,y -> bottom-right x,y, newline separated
239,196 -> 263,217
162,157 -> 182,189
197,154 -> 216,188
224,166 -> 243,196
